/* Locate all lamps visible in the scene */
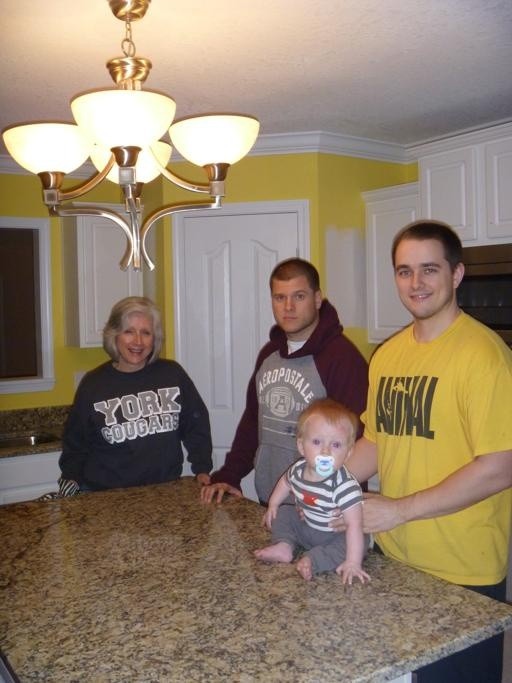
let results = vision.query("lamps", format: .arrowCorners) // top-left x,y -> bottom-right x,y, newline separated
0,0 -> 263,273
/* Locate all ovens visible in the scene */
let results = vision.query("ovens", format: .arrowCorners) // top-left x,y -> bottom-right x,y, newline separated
455,242 -> 511,347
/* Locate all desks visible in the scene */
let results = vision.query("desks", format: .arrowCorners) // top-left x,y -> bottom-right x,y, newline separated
0,474 -> 511,682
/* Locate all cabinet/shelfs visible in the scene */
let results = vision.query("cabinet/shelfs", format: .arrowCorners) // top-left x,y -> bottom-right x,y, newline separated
61,199 -> 145,349
363,181 -> 422,348
405,117 -> 512,249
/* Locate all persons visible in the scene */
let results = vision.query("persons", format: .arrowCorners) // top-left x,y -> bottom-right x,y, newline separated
254,398 -> 372,585
295,220 -> 512,683
58,297 -> 214,493
200,257 -> 369,509
